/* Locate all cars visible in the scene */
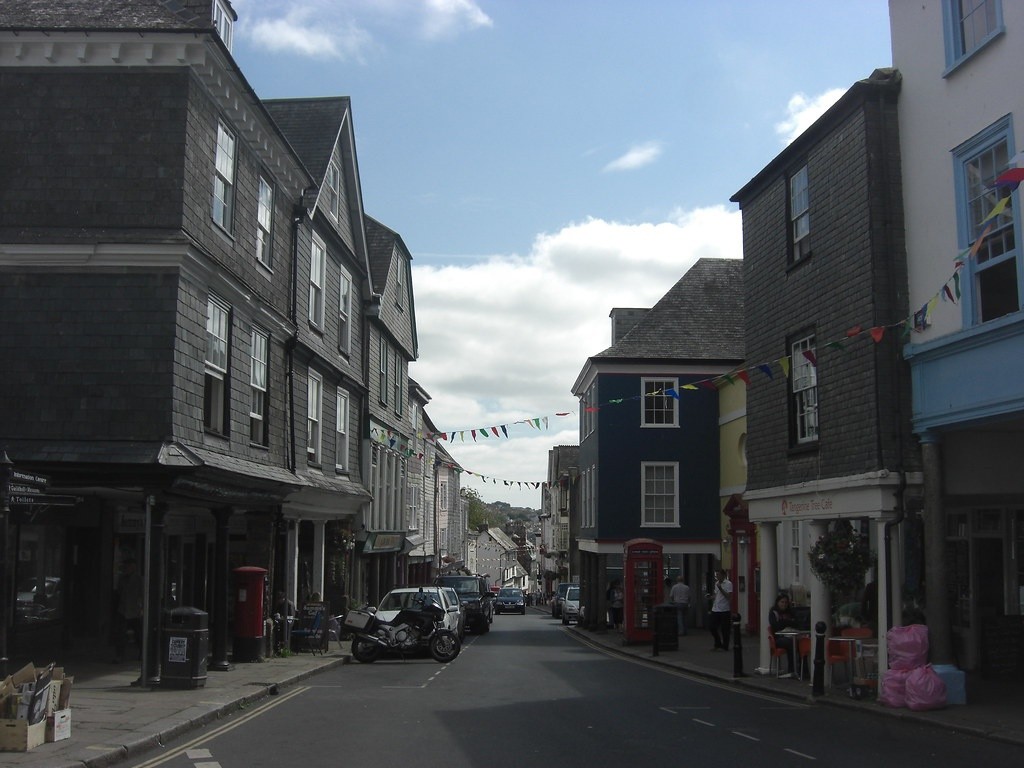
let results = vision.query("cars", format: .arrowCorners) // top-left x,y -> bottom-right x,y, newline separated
394,587 -> 469,643
495,588 -> 528,615
16,577 -> 61,625
366,588 -> 458,656
559,587 -> 579,625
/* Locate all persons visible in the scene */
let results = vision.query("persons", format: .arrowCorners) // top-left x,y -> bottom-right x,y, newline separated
768,593 -> 795,673
706,568 -> 732,651
861,581 -> 879,639
536,589 -> 542,606
273,592 -> 321,635
901,594 -> 927,627
670,575 -> 690,635
607,579 -> 624,632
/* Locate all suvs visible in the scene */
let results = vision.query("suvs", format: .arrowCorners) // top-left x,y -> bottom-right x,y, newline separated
551,583 -> 580,618
433,574 -> 495,634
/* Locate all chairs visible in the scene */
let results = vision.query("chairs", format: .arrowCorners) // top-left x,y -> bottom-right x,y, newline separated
766,625 -> 873,685
290,611 -> 324,656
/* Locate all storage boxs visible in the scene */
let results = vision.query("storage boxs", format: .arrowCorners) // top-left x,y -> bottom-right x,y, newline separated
0,661 -> 74,753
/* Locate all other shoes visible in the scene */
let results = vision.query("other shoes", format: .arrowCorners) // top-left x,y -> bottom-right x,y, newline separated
711,646 -> 726,652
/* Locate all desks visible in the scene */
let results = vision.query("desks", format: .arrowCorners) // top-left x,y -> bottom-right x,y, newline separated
829,635 -> 873,689
774,630 -> 811,681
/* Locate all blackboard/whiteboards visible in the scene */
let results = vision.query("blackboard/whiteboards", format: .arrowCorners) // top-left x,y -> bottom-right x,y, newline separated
293,598 -> 332,653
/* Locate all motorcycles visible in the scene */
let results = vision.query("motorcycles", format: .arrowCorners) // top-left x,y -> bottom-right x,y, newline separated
344,586 -> 461,664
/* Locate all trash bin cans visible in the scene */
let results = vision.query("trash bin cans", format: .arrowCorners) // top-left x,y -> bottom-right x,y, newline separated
652,603 -> 680,652
157,604 -> 210,689
